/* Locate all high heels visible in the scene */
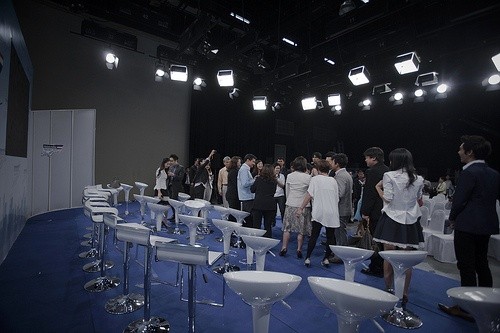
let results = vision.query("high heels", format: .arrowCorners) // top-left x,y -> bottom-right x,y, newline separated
279,248 -> 287,257
321,258 -> 329,268
304,258 -> 311,267
296,250 -> 303,259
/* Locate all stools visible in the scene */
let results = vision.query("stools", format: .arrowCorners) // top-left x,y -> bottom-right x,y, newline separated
308,245 -> 500,333
79,181 -> 302,333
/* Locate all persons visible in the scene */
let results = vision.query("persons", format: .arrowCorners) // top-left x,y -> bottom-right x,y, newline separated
436,175 -> 455,197
217,154 -> 294,254
438,136 -> 500,323
329,153 -> 353,264
372,148 -> 424,302
154,150 -> 216,222
279,156 -> 311,257
326,151 -> 337,176
296,159 -> 340,267
361,147 -> 391,278
347,167 -> 366,221
307,152 -> 322,176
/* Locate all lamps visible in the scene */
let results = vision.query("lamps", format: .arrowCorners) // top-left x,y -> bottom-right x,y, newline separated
481,52 -> 500,92
299,52 -> 450,116
217,70 -> 240,100
252,95 -> 282,112
105,45 -> 119,69
153,60 -> 207,91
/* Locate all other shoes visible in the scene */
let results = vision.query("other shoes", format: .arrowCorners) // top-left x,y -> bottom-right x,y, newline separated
329,256 -> 341,263
361,267 -> 384,278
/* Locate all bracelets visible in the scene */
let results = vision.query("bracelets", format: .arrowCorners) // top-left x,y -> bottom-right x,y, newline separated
300,207 -> 303,210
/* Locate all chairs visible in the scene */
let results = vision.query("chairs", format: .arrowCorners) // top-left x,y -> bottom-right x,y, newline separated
420,193 -> 457,265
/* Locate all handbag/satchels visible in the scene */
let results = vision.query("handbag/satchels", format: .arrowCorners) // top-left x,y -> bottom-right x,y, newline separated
358,219 -> 382,270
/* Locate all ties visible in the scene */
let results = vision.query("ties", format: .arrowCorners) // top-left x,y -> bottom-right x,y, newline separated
168,166 -> 175,185
